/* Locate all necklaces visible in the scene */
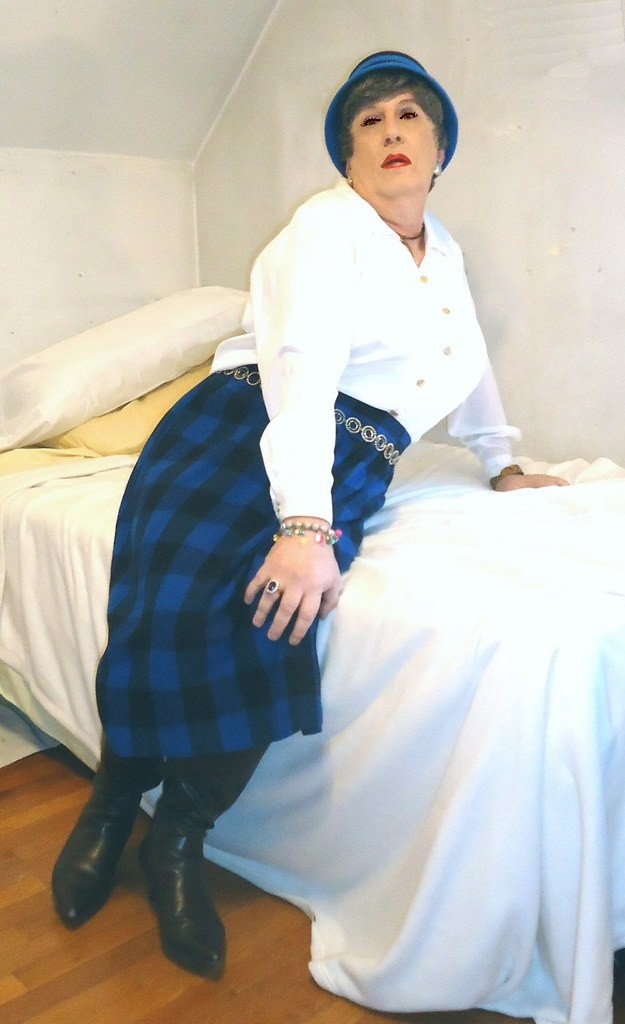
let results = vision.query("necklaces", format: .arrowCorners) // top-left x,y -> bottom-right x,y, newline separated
385,223 -> 425,254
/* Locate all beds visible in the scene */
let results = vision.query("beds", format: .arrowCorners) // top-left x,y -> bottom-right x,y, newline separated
0,445 -> 625,1024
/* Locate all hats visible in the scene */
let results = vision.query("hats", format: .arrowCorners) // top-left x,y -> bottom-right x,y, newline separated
324,51 -> 458,178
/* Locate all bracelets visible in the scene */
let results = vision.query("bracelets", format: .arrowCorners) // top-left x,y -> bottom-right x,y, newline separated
489,465 -> 523,493
272,519 -> 343,546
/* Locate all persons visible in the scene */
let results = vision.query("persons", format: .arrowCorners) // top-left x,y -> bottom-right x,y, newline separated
52,49 -> 571,984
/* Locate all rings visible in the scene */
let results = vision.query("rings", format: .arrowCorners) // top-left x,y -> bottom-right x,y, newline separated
267,582 -> 280,596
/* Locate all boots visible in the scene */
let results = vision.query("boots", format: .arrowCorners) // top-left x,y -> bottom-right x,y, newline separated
136,743 -> 268,977
51,737 -> 161,930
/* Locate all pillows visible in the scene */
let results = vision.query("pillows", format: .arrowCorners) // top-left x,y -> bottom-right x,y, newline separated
1,286 -> 250,454
35,356 -> 213,454
